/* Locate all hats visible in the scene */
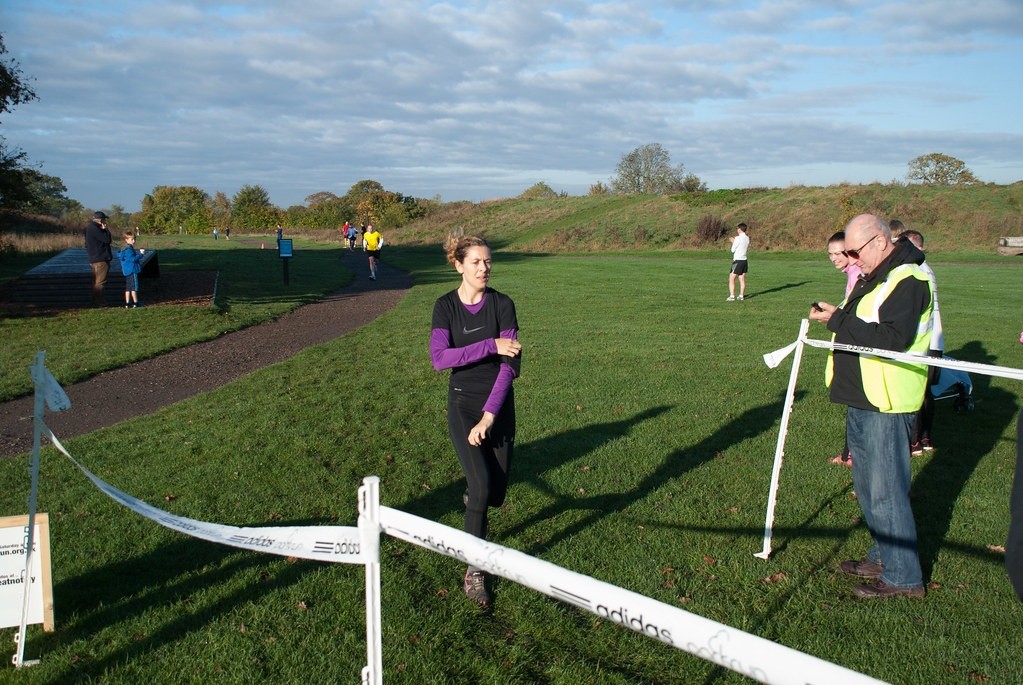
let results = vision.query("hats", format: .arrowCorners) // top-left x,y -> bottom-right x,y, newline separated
92,212 -> 109,219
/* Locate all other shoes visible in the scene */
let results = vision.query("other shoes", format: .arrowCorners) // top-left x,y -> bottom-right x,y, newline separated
368,276 -> 376,281
736,297 -> 744,300
125,303 -> 142,308
726,297 -> 735,300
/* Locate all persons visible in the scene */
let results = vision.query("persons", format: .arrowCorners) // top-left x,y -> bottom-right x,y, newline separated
810,212 -> 934,600
343,221 -> 358,253
116,230 -> 145,310
274,224 -> 283,249
726,223 -> 750,300
84,211 -> 113,307
363,224 -> 384,280
360,222 -> 367,247
828,219 -> 943,467
213,226 -> 218,240
224,226 -> 230,240
428,228 -> 522,605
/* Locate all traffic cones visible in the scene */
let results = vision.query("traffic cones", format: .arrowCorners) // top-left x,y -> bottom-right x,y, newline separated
261,243 -> 264,250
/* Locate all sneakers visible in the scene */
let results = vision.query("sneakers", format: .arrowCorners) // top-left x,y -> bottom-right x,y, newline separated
910,441 -> 923,455
463,569 -> 491,605
921,437 -> 933,449
841,558 -> 882,578
463,487 -> 469,504
828,455 -> 852,467
852,580 -> 926,599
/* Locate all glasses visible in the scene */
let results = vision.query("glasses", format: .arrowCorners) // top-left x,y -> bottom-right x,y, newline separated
841,234 -> 880,259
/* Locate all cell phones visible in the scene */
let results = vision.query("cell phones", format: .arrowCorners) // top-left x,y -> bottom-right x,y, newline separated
811,303 -> 824,312
